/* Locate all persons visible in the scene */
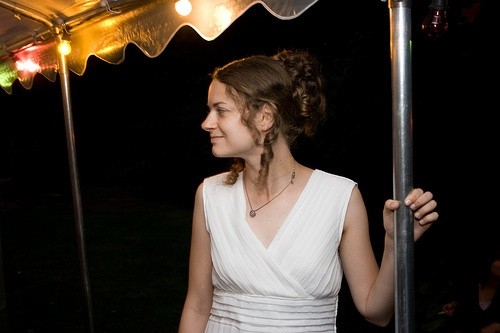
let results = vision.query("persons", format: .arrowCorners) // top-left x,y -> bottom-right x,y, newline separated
442,258 -> 500,333
178,50 -> 438,333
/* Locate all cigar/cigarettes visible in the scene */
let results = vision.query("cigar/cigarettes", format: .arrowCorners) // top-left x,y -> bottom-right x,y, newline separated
439,311 -> 449,315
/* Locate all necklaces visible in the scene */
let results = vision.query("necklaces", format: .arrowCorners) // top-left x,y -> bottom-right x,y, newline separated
243,160 -> 296,217
